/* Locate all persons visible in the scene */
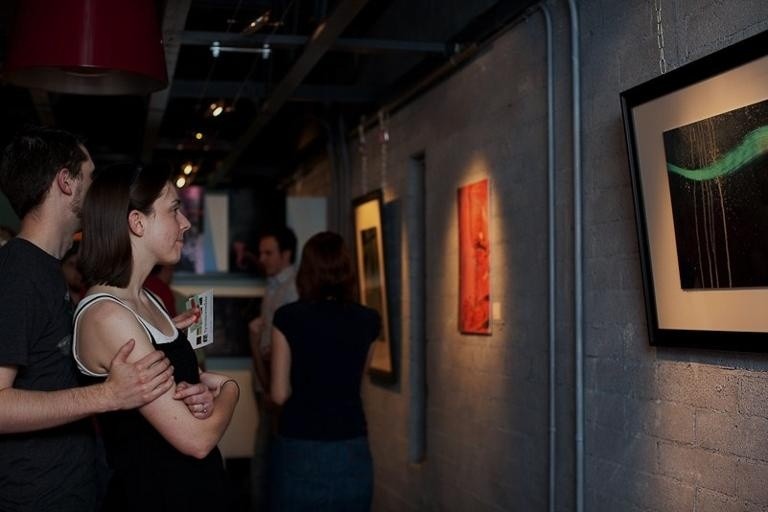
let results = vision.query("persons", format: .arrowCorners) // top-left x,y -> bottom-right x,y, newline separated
261,230 -> 383,511
243,225 -> 301,512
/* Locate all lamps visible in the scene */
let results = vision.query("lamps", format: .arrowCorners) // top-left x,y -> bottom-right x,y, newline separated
0,0 -> 171,102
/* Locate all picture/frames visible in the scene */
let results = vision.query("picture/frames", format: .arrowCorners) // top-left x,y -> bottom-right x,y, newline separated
349,187 -> 398,380
617,28 -> 768,352
